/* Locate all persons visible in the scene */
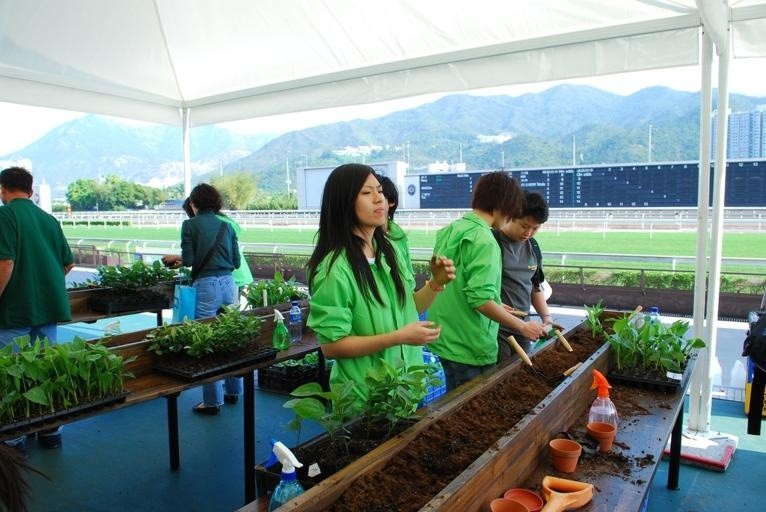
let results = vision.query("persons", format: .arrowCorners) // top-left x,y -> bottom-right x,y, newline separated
375,172 -> 416,293
180,183 -> 244,414
492,192 -> 567,366
303,163 -> 458,416
421,170 -> 549,395
0,167 -> 75,451
160,195 -> 253,312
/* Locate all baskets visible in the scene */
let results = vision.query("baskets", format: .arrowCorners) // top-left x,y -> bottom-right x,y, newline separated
256,364 -> 331,394
421,352 -> 449,406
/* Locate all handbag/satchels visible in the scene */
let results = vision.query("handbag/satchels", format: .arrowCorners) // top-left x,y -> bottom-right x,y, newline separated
172,285 -> 196,325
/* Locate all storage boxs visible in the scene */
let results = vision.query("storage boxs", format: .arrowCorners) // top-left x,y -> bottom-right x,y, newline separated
258,364 -> 319,394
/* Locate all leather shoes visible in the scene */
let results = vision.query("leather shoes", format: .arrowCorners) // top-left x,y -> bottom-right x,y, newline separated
192,402 -> 221,414
224,395 -> 238,404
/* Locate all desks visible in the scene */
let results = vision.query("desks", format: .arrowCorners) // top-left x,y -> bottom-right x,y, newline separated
234,340 -> 700,512
0,325 -> 329,505
55,295 -> 173,326
748,311 -> 766,435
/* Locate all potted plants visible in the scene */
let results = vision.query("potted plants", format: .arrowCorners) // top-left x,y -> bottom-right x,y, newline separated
600,311 -> 700,393
0,334 -> 139,435
87,260 -> 170,314
253,357 -> 444,500
144,304 -> 282,382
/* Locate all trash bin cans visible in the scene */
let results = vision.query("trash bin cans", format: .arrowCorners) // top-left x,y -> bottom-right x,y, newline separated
745,311 -> 766,417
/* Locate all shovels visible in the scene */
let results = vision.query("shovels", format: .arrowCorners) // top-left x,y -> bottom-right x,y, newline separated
510,310 -> 551,317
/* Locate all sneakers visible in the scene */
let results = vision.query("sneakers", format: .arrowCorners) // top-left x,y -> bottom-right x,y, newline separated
5,437 -> 24,446
38,428 -> 62,448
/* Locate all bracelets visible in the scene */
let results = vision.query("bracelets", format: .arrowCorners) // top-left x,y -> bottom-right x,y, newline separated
427,278 -> 445,293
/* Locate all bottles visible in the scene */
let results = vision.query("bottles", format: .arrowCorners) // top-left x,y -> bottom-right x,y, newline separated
289,301 -> 302,344
648,307 -> 661,325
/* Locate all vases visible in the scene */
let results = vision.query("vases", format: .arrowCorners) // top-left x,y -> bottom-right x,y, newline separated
586,422 -> 616,452
548,438 -> 582,473
490,498 -> 529,512
